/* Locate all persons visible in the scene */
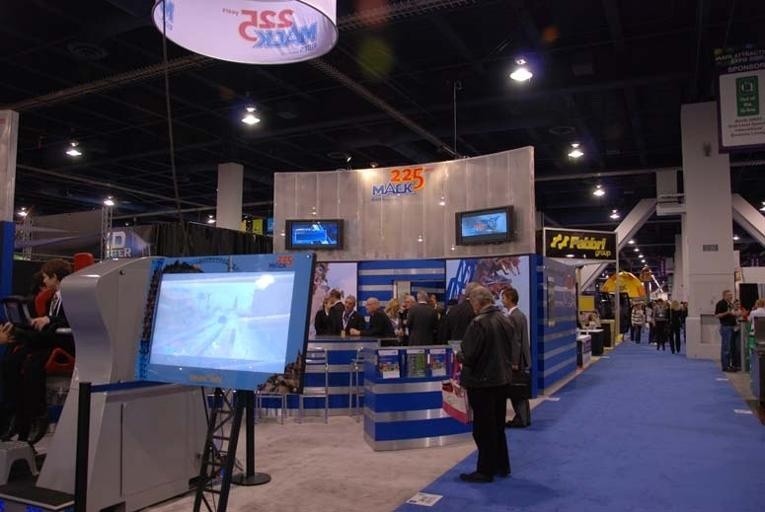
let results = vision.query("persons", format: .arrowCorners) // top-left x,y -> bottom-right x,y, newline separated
0,259 -> 71,447
27,272 -> 47,319
501,289 -> 531,427
316,288 -> 444,347
443,282 -> 479,341
453,286 -> 515,484
587,314 -> 601,330
714,290 -> 765,372
628,297 -> 688,355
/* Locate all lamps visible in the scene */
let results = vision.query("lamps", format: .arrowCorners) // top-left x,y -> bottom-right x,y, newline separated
65,139 -> 83,157
592,181 -> 606,199
102,193 -> 117,206
14,206 -> 31,217
509,54 -> 534,83
566,140 -> 584,162
239,95 -> 259,127
206,213 -> 215,223
609,208 -> 664,293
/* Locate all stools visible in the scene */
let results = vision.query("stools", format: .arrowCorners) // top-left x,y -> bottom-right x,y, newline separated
1,440 -> 40,485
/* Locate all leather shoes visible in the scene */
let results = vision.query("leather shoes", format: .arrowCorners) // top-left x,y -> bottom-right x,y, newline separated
506,420 -> 532,429
22,411 -> 51,446
461,471 -> 496,485
0,410 -> 22,442
488,470 -> 511,477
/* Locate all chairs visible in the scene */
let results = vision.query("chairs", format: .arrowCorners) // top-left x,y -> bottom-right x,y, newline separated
349,345 -> 374,416
299,347 -> 329,423
258,392 -> 297,423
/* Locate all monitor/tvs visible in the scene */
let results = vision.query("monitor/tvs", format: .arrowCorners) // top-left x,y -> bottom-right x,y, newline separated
132,250 -> 317,396
285,218 -> 344,250
455,205 -> 513,246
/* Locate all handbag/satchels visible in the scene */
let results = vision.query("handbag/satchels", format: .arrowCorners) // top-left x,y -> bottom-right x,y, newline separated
441,380 -> 474,425
